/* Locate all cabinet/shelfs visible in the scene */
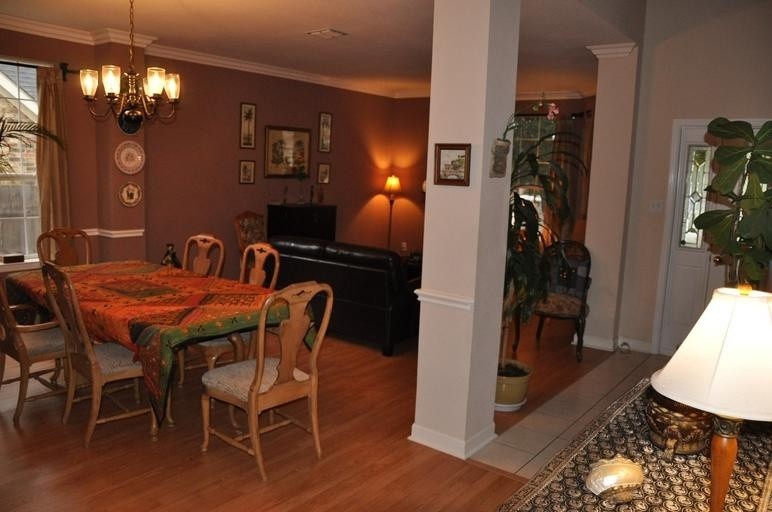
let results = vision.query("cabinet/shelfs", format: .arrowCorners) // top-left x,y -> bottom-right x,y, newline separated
265,203 -> 338,243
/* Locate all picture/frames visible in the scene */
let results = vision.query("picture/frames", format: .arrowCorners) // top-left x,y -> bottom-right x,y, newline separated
317,162 -> 332,186
239,102 -> 257,151
239,159 -> 256,186
433,141 -> 472,187
264,123 -> 312,180
317,111 -> 334,154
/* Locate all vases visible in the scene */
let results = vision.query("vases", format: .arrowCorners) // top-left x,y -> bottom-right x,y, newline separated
487,137 -> 512,179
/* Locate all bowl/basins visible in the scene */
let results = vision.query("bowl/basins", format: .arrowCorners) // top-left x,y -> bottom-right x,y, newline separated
638,383 -> 723,462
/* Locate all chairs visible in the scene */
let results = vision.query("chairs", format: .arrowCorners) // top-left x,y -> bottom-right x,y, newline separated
202,280 -> 334,479
183,234 -> 225,277
508,240 -> 593,365
36,228 -> 91,267
240,243 -> 280,289
0,261 -> 160,448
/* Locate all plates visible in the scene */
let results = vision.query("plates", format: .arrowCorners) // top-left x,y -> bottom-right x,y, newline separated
113,140 -> 149,177
117,181 -> 144,208
116,107 -> 144,137
586,457 -> 645,500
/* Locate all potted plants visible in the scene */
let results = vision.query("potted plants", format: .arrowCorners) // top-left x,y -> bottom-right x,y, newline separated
494,130 -> 593,411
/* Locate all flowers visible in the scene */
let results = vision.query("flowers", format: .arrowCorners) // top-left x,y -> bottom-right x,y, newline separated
501,90 -> 561,139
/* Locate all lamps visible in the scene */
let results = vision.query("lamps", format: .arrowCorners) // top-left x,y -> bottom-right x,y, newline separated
646,284 -> 771,512
78,0 -> 184,129
383,174 -> 402,250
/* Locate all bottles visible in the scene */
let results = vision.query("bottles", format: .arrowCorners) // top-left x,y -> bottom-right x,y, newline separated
159,243 -> 182,270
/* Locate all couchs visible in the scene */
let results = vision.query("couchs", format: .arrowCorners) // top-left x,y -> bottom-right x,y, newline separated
269,235 -> 423,359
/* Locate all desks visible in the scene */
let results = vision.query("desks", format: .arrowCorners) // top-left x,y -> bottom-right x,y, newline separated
0,259 -> 311,442
489,375 -> 772,512
